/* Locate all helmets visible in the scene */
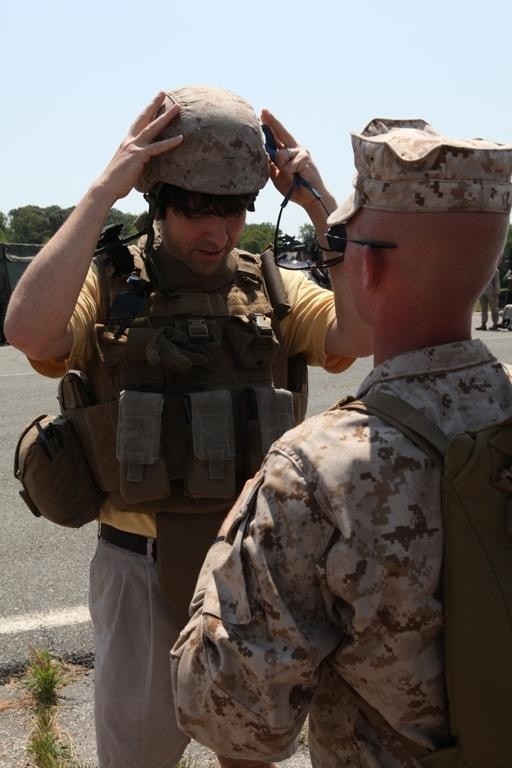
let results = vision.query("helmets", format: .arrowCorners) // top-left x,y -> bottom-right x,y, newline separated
132,84 -> 269,194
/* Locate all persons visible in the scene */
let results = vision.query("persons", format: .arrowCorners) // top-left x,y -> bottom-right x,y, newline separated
166,117 -> 511,767
3,90 -> 377,768
474,267 -> 500,331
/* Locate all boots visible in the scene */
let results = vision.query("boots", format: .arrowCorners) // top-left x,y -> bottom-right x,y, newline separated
474,319 -> 510,331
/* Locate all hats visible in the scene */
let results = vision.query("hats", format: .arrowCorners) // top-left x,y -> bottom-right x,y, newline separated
325,117 -> 511,227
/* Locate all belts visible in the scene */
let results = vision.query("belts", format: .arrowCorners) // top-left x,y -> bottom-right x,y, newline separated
97,523 -> 159,560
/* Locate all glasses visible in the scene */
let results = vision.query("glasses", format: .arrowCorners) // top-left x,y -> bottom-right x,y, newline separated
324,224 -> 397,256
275,174 -> 344,270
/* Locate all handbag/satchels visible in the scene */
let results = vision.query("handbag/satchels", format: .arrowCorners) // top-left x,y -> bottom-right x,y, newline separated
13,410 -> 101,530
